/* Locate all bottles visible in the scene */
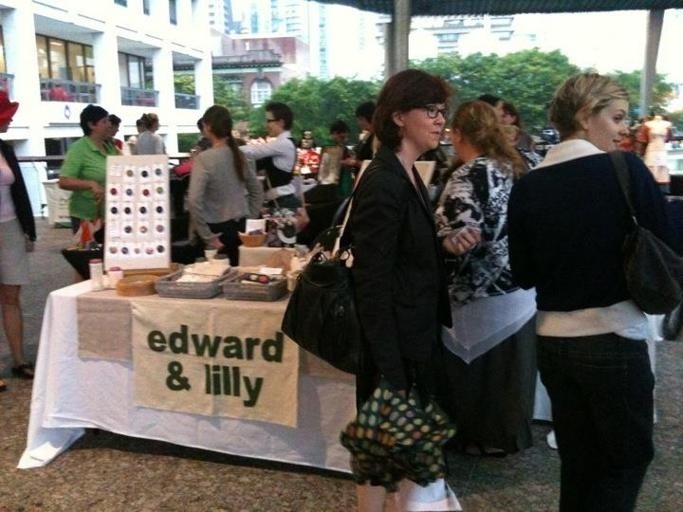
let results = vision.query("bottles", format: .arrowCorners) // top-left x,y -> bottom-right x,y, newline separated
88,258 -> 104,292
107,266 -> 122,290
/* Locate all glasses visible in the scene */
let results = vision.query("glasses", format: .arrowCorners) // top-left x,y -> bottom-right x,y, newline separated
422,105 -> 448,118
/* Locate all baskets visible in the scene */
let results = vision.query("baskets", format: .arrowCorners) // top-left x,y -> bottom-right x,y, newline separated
155,270 -> 286,301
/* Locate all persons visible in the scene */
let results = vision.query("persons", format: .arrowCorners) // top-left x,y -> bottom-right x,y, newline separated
1,88 -> 41,394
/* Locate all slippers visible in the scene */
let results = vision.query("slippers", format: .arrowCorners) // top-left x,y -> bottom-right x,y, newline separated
12,362 -> 34,378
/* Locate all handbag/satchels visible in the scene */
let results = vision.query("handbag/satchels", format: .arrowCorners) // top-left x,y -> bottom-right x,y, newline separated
282,252 -> 365,375
622,228 -> 683,314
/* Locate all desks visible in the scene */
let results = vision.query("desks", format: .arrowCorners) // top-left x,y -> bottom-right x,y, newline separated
61,238 -> 199,278
237,244 -> 296,272
531,310 -> 668,432
46,265 -> 358,477
